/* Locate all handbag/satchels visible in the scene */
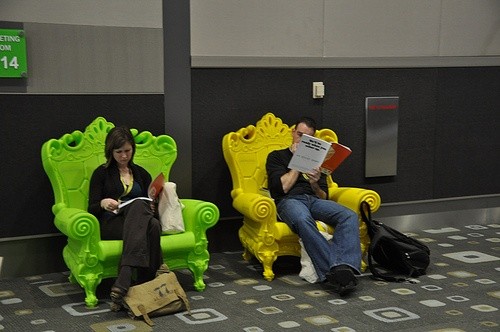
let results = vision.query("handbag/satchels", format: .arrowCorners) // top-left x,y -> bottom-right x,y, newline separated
119,263 -> 192,328
158,181 -> 187,235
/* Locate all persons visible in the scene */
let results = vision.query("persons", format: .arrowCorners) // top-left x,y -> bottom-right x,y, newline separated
267,117 -> 362,296
87,127 -> 162,305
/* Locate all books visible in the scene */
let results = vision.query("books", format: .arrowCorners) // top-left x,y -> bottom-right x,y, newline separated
111,173 -> 165,214
288,133 -> 352,176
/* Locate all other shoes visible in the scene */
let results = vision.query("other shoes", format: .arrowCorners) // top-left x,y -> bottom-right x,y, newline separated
334,284 -> 349,297
333,265 -> 357,288
110,282 -> 130,302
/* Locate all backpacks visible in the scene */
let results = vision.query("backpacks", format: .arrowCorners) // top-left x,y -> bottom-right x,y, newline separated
359,200 -> 431,284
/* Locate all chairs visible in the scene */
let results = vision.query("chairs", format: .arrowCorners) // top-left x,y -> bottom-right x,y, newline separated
222,113 -> 380,282
40,118 -> 221,310
